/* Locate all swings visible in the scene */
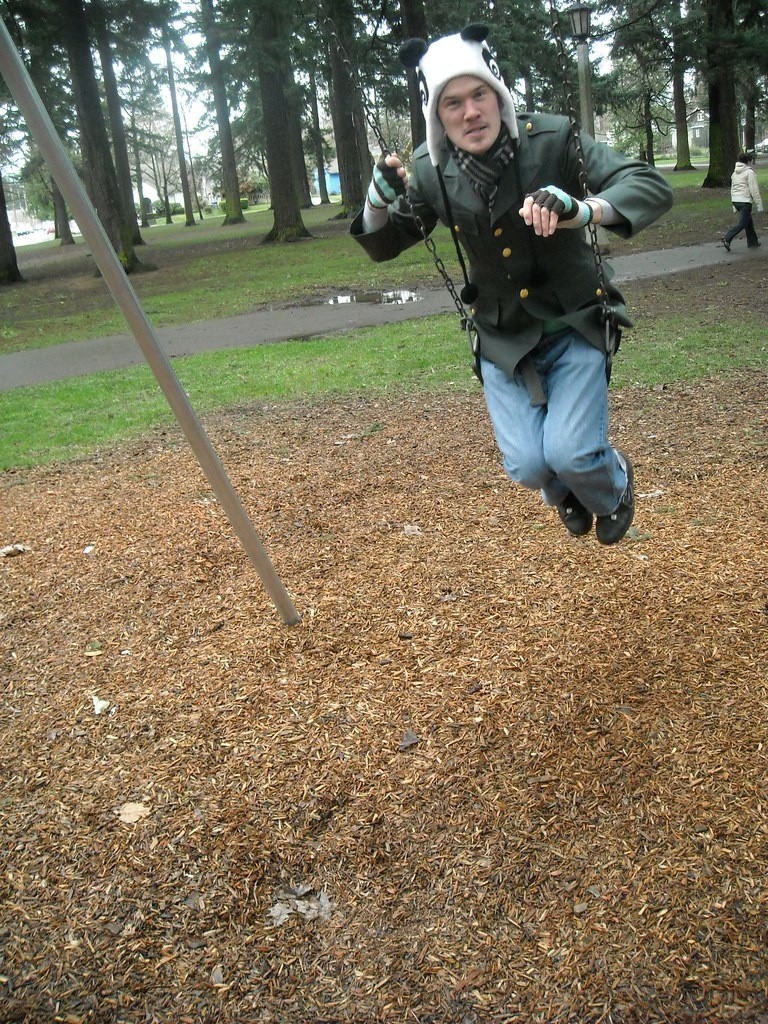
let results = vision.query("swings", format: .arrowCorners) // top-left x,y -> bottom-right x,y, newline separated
314,0 -> 616,388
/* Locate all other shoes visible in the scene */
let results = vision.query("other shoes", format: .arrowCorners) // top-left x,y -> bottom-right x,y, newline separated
721,237 -> 731,252
747,242 -> 762,248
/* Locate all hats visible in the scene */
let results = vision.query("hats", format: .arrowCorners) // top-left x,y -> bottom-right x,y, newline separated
400,22 -> 520,168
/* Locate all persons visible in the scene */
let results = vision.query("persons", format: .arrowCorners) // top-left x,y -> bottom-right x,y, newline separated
721,154 -> 764,252
348,21 -> 675,544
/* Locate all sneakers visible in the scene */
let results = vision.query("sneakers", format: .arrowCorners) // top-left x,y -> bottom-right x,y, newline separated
554,452 -> 636,546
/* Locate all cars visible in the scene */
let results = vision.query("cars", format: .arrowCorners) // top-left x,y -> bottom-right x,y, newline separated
755,139 -> 768,153
16,220 -> 55,236
